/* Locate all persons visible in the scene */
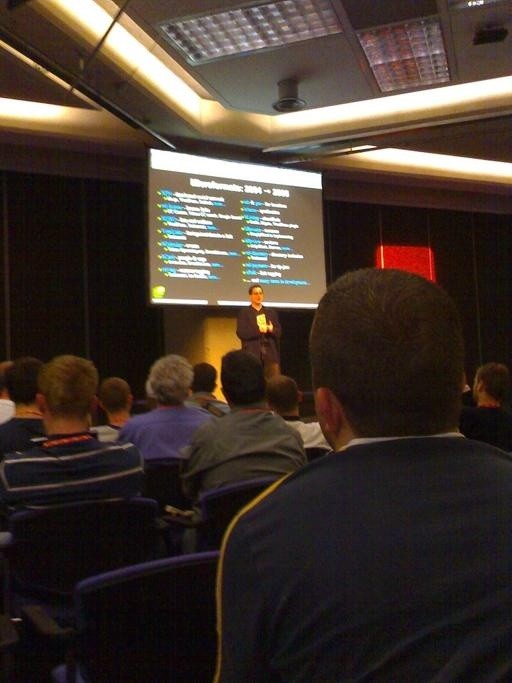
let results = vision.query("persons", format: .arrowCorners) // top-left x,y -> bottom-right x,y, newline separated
209,265 -> 512,683
0,349 -> 512,683
234,283 -> 284,380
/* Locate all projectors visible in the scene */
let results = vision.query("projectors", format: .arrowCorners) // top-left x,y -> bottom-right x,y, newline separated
473,28 -> 508,45
